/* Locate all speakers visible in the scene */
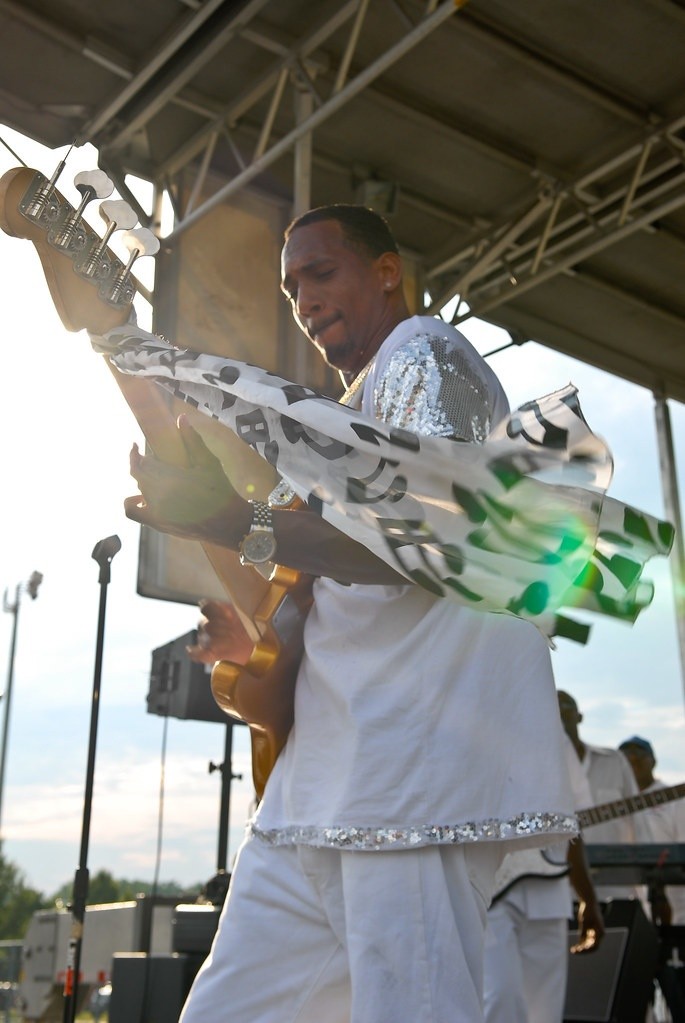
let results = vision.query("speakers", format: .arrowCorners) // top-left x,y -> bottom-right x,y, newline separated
146,629 -> 248,725
563,925 -> 662,1023
107,953 -> 209,1023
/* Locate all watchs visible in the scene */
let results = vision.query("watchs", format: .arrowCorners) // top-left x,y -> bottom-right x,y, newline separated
239,500 -> 275,566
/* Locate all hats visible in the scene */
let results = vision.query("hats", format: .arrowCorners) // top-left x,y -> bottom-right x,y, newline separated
618,736 -> 653,754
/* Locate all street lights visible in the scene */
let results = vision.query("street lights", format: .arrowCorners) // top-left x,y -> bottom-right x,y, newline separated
61,535 -> 122,1022
0,570 -> 43,816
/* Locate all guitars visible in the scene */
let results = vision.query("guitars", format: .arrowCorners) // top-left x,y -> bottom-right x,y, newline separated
0,141 -> 315,802
575,783 -> 685,829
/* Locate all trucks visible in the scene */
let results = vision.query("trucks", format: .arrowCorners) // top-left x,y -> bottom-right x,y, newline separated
20,901 -> 174,1023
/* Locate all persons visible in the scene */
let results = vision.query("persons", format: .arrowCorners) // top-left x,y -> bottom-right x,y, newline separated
482,832 -> 605,1023
125,205 -> 579,1023
555,692 -> 685,1023
